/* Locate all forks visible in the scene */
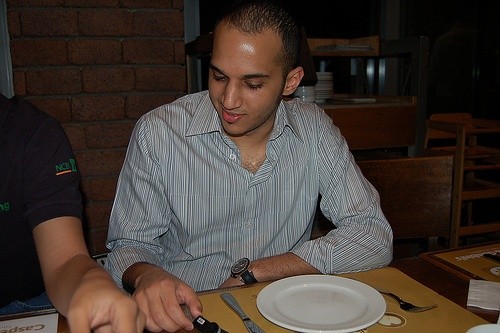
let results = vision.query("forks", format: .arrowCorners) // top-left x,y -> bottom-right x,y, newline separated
381,291 -> 438,312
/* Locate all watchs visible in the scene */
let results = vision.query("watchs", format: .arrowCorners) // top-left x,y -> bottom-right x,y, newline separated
230,257 -> 258,285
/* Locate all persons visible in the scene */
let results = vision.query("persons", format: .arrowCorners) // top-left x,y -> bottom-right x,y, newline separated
102,0 -> 393,333
0,91 -> 146,333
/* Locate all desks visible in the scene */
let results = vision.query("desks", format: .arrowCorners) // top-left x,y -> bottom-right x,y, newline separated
0,240 -> 500,333
316,93 -> 418,151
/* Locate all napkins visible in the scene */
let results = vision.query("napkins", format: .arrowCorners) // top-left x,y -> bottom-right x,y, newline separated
467,279 -> 500,311
0,313 -> 59,333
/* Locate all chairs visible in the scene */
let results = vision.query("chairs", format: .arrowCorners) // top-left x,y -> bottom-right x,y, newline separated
356,155 -> 453,256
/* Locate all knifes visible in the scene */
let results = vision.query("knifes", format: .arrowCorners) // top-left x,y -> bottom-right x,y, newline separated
220,292 -> 265,333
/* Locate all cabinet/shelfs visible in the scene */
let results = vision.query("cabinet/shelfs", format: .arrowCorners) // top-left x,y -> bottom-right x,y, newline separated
426,113 -> 500,249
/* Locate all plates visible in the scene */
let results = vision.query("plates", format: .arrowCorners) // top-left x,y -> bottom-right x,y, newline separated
256,274 -> 387,333
465,323 -> 500,333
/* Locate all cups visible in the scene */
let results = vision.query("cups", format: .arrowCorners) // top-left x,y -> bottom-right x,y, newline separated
314,72 -> 334,100
292,86 -> 315,103
